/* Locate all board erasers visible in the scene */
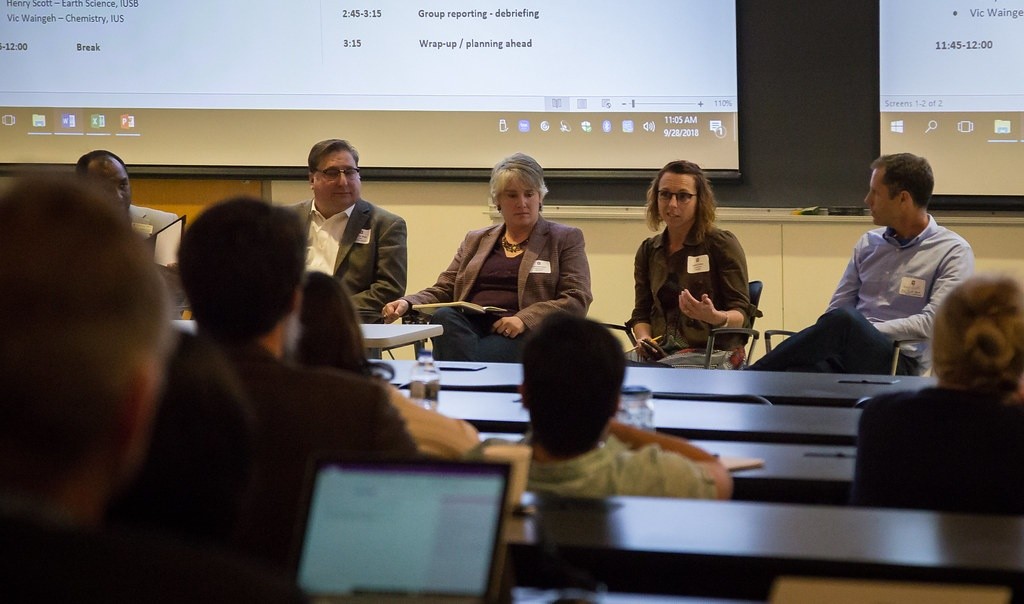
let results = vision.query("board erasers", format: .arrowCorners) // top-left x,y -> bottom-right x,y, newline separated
828,205 -> 865,216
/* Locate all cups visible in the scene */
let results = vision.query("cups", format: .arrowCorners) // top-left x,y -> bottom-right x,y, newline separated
613,385 -> 656,435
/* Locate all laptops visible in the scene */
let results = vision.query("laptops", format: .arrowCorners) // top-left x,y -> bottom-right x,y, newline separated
293,457 -> 517,603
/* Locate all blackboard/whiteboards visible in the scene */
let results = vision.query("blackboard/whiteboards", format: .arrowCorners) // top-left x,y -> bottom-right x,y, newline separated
482,0 -> 1024,225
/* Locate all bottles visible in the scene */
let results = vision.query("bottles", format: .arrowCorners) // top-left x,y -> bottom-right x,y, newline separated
410,349 -> 438,412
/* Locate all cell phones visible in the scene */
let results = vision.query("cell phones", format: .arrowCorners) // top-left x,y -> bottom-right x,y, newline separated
641,341 -> 660,361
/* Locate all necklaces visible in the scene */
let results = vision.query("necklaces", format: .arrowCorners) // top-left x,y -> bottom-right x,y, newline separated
502,235 -> 529,252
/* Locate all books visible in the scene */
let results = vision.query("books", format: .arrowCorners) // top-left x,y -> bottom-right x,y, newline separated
412,301 -> 507,317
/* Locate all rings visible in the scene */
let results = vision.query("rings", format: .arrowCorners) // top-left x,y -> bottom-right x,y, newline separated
384,315 -> 387,318
505,330 -> 509,334
685,310 -> 689,313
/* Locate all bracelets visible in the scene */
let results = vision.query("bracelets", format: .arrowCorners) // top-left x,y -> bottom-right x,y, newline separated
718,310 -> 729,328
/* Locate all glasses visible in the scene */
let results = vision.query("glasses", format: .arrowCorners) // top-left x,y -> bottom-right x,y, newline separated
316,167 -> 360,181
656,188 -> 697,204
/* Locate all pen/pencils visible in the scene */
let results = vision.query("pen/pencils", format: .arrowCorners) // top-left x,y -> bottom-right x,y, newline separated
652,333 -> 667,341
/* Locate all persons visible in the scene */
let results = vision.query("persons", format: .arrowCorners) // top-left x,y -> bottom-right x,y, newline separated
624,160 -> 751,369
471,312 -> 731,501
1,169 -> 174,604
107,195 -> 416,571
382,152 -> 594,363
75,150 -> 186,320
850,276 -> 1024,518
742,153 -> 976,377
294,139 -> 408,324
283,271 -> 478,457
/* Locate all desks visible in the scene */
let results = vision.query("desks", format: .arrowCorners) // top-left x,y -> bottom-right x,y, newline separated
371,358 -> 1024,604
169,320 -> 444,360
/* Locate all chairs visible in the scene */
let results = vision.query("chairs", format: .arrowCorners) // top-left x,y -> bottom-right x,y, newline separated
595,280 -> 935,376
